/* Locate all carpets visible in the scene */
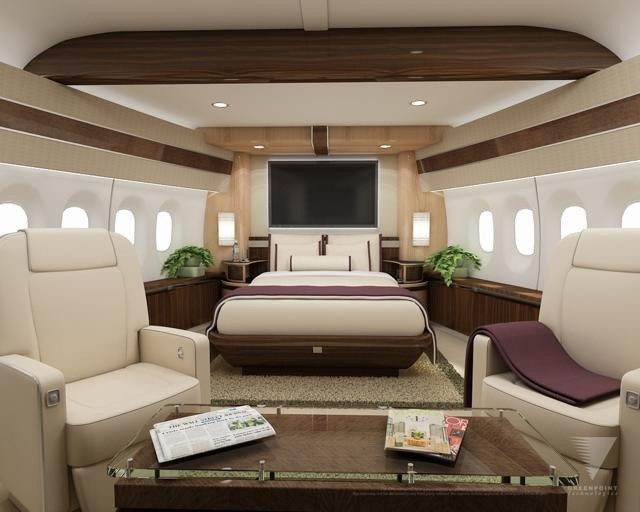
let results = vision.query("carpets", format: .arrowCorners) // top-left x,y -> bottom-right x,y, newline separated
211,345 -> 466,406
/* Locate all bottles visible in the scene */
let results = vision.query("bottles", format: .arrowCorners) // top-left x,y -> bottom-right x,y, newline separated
232,241 -> 240,262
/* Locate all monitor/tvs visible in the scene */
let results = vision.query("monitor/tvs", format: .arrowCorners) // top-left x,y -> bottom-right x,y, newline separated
267,160 -> 378,228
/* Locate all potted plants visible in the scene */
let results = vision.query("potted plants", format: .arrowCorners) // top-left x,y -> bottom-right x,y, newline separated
160,246 -> 216,280
423,246 -> 482,288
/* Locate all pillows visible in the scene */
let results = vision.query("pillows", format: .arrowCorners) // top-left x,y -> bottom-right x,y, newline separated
275,241 -> 371,271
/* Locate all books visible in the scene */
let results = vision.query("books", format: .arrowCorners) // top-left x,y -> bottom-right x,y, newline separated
428,414 -> 469,465
383,406 -> 453,456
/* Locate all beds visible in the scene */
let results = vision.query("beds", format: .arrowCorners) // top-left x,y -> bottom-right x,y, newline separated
207,234 -> 432,376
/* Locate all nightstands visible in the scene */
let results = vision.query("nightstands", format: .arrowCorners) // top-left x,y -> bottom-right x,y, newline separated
398,280 -> 429,319
220,280 -> 250,298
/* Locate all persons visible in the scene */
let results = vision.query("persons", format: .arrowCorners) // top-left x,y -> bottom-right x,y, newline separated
235,417 -> 265,430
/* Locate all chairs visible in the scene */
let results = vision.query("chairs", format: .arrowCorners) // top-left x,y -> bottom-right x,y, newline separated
0,227 -> 209,512
471,229 -> 640,512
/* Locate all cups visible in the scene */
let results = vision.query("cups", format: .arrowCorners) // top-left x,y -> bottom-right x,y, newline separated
241,248 -> 248,261
